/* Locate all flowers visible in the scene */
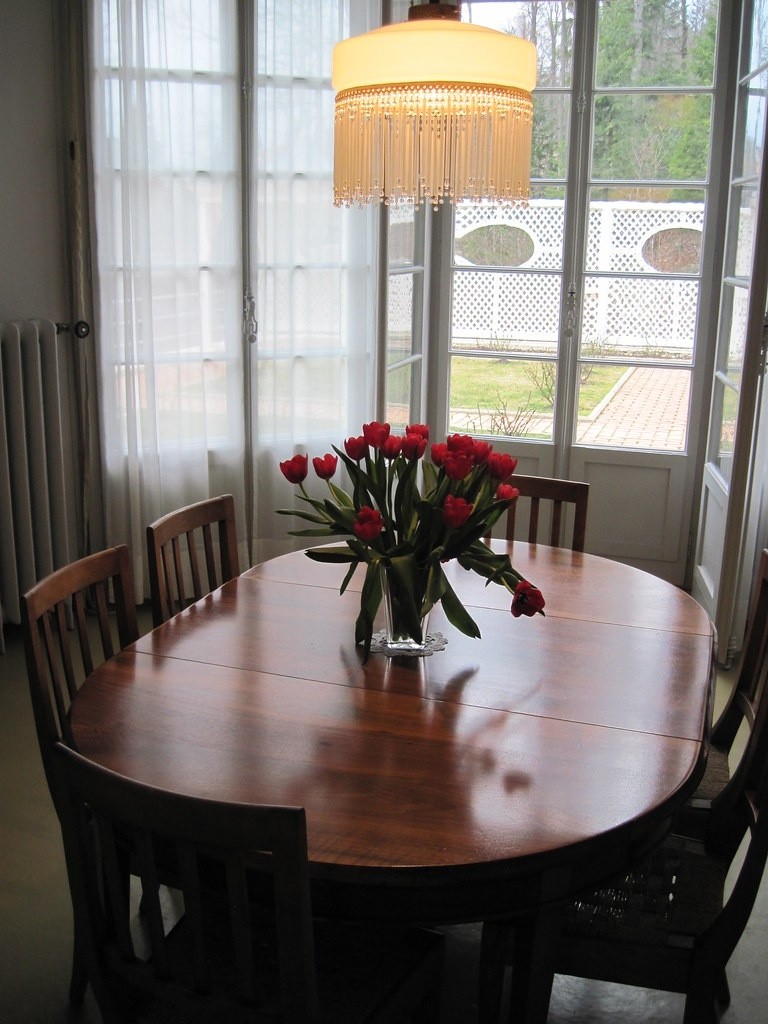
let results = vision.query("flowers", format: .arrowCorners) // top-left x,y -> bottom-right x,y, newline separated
275,421 -> 547,668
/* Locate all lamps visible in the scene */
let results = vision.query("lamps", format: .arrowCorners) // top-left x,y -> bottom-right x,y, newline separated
332,0 -> 537,212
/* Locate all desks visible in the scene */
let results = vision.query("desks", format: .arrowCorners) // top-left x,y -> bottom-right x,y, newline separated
64,537 -> 714,1024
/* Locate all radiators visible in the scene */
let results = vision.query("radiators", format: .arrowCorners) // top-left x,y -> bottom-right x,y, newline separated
0,320 -> 90,627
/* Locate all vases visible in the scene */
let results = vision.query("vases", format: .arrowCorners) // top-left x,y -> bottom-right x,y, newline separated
379,561 -> 433,650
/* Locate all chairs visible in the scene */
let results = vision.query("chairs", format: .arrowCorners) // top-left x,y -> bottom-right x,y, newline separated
482,549 -> 768,1024
19,543 -> 137,1024
146,494 -> 240,629
56,741 -> 447,1024
484,473 -> 590,552
